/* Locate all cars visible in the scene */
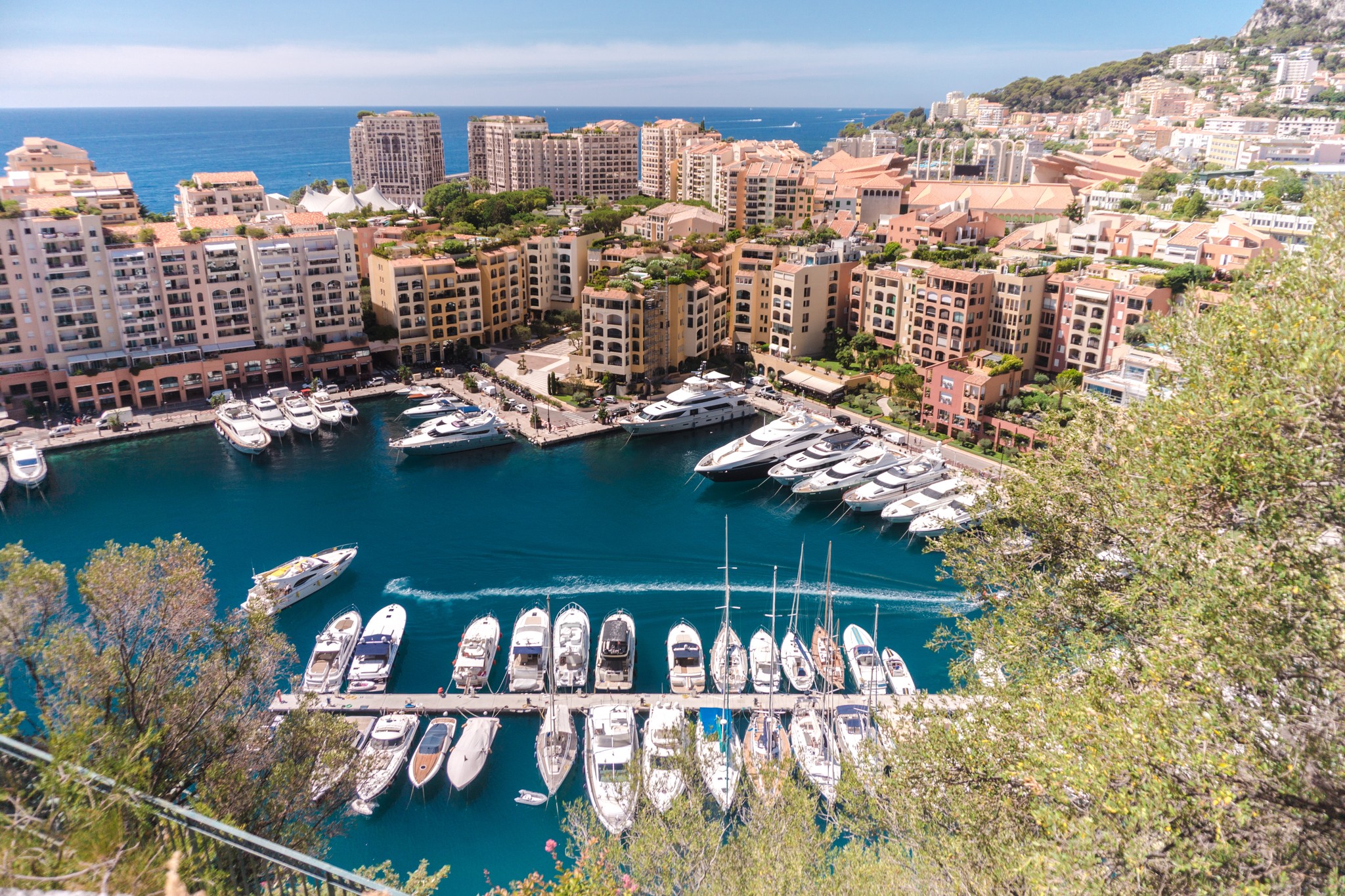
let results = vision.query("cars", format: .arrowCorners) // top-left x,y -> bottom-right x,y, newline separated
0,437 -> 5,446
501,398 -> 529,414
584,395 -> 649,421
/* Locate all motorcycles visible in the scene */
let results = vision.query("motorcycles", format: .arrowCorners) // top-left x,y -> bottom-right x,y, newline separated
472,367 -> 537,401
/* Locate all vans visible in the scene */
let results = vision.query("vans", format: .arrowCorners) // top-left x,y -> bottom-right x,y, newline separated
49,424 -> 72,438
832,415 -> 851,426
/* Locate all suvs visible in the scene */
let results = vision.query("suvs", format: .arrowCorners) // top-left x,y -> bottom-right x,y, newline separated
365,376 -> 385,388
298,375 -> 349,397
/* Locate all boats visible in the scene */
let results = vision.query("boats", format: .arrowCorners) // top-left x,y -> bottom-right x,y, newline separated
693,390 -> 846,482
767,420 -> 1040,556
240,542 -> 359,620
615,361 -> 759,438
388,382 -> 515,457
214,386 -> 359,456
267,513 -> 1007,835
0,439 -> 48,495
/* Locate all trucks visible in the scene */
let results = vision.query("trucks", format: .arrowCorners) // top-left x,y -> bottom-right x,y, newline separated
95,406 -> 133,431
748,376 -> 783,400
206,389 -> 233,405
474,380 -> 498,397
265,386 -> 289,400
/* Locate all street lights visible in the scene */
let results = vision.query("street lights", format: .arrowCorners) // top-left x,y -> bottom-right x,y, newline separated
644,376 -> 652,405
43,401 -> 50,430
907,413 -> 919,450
359,372 -> 362,387
